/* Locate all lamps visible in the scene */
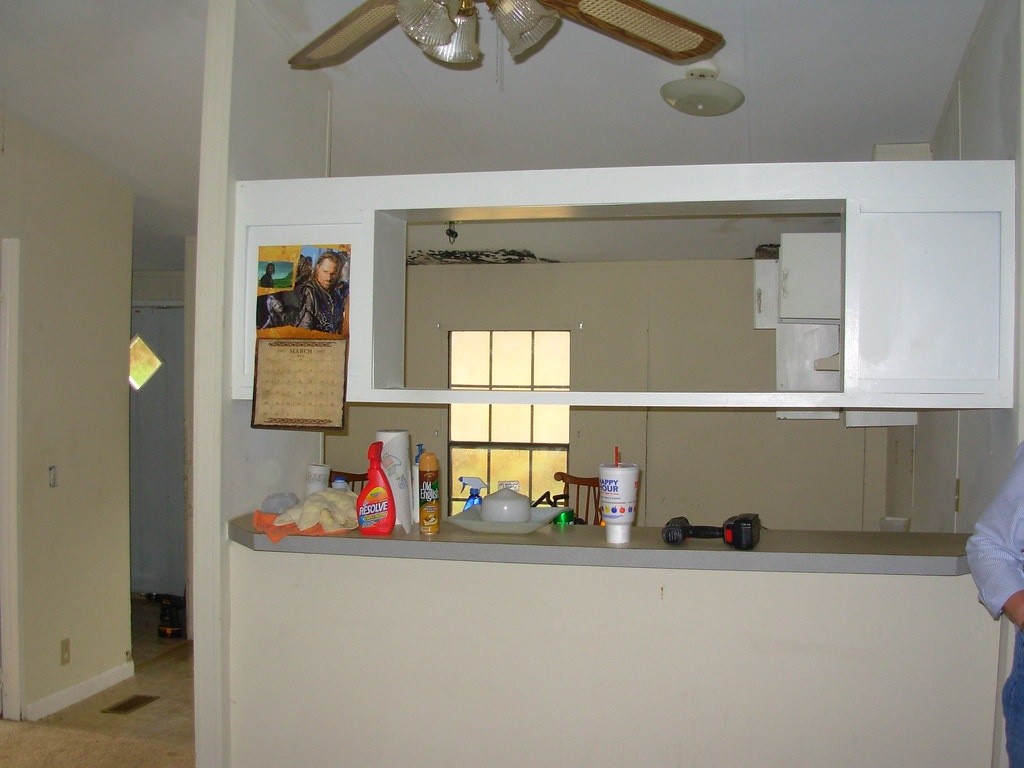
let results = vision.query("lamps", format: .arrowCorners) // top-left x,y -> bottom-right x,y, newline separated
395,0 -> 561,65
659,76 -> 746,117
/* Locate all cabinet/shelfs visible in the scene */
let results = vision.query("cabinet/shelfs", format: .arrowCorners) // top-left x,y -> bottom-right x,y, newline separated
227,159 -> 1015,428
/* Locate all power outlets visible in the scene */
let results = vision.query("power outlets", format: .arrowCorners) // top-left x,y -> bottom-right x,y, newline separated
61,638 -> 70,665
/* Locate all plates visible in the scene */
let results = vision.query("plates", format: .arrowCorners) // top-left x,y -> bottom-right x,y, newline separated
440,503 -> 568,535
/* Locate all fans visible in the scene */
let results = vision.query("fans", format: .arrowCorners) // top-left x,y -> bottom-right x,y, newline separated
286,0 -> 724,68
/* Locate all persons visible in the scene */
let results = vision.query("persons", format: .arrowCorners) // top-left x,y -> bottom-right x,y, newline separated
260,262 -> 276,289
964,439 -> 1024,768
258,249 -> 350,334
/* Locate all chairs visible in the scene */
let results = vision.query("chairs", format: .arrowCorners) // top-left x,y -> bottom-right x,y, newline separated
554,471 -> 607,527
328,469 -> 372,498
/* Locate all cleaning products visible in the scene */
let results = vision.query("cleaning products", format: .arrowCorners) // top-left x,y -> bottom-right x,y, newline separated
413,442 -> 443,536
356,439 -> 396,537
459,476 -> 487,509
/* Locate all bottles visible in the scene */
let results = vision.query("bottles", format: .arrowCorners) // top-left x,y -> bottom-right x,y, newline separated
419,453 -> 443,536
552,508 -> 575,527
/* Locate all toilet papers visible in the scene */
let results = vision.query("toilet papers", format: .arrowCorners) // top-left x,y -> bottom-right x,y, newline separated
303,461 -> 331,501
375,425 -> 415,533
